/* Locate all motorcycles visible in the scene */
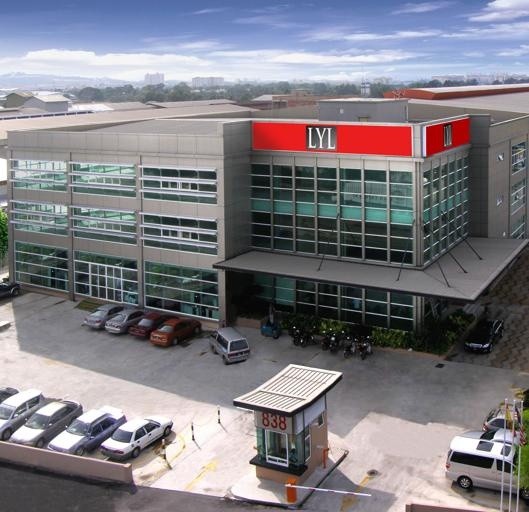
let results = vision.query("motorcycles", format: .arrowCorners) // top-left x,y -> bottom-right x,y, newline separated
328,298 -> 406,326
290,312 -> 373,360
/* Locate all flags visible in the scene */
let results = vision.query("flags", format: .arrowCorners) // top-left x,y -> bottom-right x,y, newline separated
506,404 -> 517,438
514,406 -> 527,446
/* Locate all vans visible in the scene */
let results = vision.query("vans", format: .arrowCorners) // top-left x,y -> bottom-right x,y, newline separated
209,326 -> 250,366
0,389 -> 47,440
483,407 -> 522,432
444,435 -> 529,500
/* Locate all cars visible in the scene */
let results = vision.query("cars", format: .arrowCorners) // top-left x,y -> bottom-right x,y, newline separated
0,386 -> 19,403
8,399 -> 85,447
48,404 -> 126,456
84,304 -> 127,329
0,282 -> 21,299
111,280 -> 216,314
151,318 -> 202,347
127,312 -> 178,339
99,414 -> 174,461
460,427 -> 525,445
105,309 -> 149,335
464,318 -> 505,354
